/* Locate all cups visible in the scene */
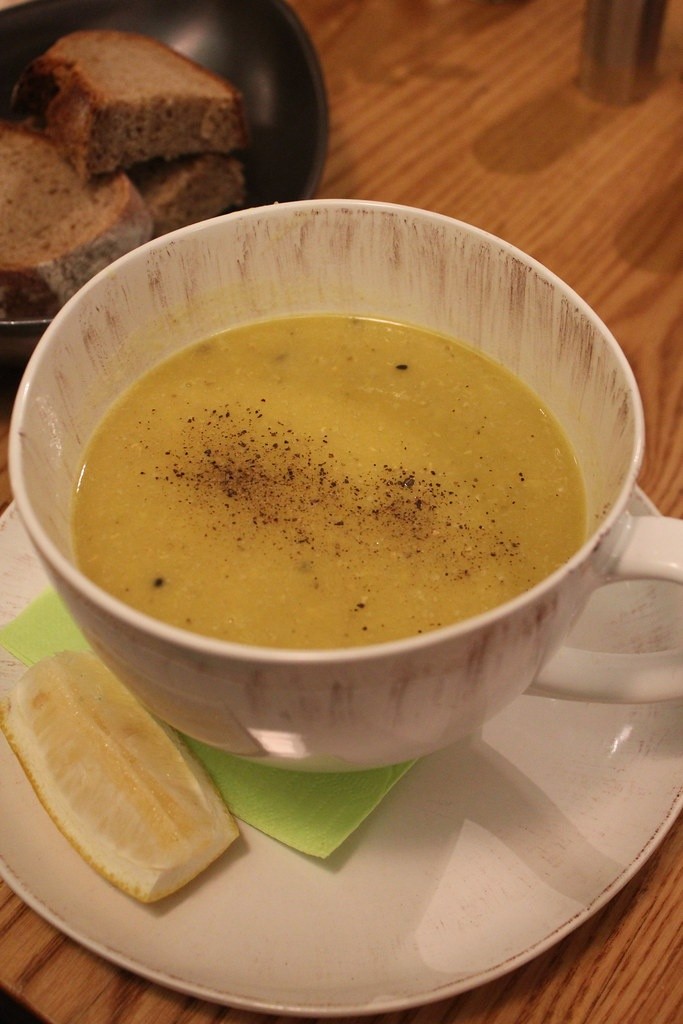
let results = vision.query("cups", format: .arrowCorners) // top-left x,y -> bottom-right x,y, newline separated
8,197 -> 683,773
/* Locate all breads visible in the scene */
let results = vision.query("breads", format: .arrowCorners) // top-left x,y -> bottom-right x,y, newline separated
0,28 -> 248,322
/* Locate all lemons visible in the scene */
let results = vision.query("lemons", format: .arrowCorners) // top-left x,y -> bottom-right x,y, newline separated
0,648 -> 241,903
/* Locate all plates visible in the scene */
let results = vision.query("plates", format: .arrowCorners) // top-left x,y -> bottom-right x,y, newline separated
0,1 -> 328,378
0,483 -> 683,1017
